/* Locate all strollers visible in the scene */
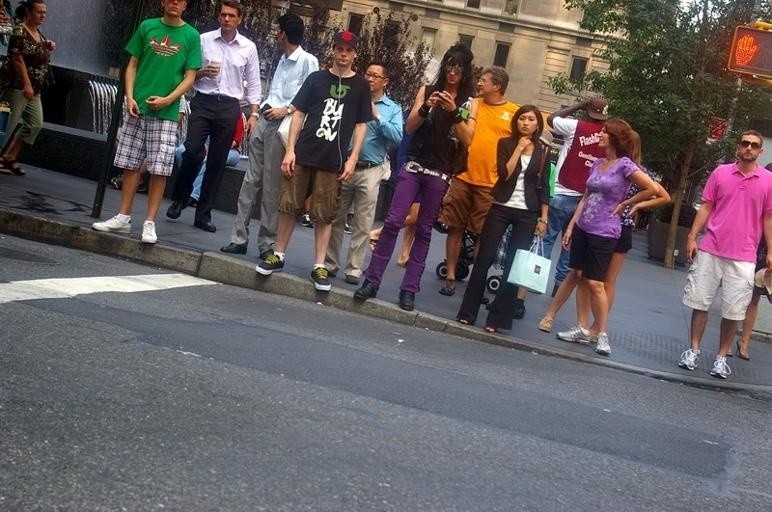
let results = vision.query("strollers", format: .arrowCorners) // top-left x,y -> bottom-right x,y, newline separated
433,223 -> 504,294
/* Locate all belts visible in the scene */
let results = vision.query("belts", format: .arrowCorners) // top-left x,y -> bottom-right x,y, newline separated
405,160 -> 453,184
195,90 -> 238,104
353,160 -> 385,169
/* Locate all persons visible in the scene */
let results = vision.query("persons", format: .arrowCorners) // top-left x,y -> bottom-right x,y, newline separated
677,129 -> 772,379
299,172 -> 353,235
354,43 -> 474,311
0,0 -> 56,177
538,129 -> 670,344
369,184 -> 424,268
512,288 -> 527,320
556,119 -> 660,355
91,1 -> 203,245
167,1 -> 262,233
174,107 -> 244,211
725,163 -> 772,360
535,97 -> 609,298
456,106 -> 551,334
220,13 -> 318,265
324,61 -> 403,285
437,66 -> 520,305
255,32 -> 374,292
109,95 -> 186,194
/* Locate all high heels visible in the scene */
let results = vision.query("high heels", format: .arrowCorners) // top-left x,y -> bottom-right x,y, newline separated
736,338 -> 750,361
727,350 -> 734,356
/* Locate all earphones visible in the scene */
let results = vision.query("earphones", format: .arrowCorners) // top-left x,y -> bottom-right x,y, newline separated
333,54 -> 358,171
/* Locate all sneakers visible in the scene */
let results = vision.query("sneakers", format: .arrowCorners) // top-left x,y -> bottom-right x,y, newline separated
678,350 -> 701,371
595,333 -> 612,355
329,266 -> 340,277
438,276 -> 456,295
91,212 -> 132,235
309,266 -> 332,292
557,325 -> 591,345
709,353 -> 732,379
140,220 -> 158,244
300,214 -> 313,228
345,275 -> 359,285
344,223 -> 353,234
513,305 -> 526,319
480,290 -> 490,304
255,253 -> 285,277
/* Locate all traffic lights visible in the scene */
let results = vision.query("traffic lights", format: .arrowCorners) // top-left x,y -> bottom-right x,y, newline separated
729,27 -> 768,78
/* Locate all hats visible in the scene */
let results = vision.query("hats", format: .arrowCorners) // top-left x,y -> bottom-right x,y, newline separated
586,98 -> 609,122
754,267 -> 772,305
331,29 -> 360,53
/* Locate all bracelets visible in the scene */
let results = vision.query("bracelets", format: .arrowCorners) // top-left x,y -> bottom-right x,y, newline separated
537,217 -> 547,226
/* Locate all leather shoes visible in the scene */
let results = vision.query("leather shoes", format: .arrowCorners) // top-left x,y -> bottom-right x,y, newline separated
167,201 -> 188,220
193,215 -> 217,233
537,317 -> 554,332
397,289 -> 415,310
258,249 -> 274,260
218,243 -> 246,256
355,280 -> 379,301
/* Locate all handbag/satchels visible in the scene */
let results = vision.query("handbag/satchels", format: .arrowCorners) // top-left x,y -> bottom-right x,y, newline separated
530,146 -> 551,192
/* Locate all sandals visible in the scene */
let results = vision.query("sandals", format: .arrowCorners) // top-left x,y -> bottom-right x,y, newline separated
0,155 -> 27,176
110,173 -> 123,190
139,183 -> 147,193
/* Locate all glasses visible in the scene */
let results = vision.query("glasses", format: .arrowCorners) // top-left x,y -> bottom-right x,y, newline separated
364,71 -> 387,80
443,64 -> 466,78
738,139 -> 762,150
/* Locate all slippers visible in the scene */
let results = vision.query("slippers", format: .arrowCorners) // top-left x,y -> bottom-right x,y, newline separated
368,238 -> 379,253
398,257 -> 410,268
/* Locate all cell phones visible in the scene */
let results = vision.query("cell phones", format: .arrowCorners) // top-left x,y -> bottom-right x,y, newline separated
261,104 -> 271,115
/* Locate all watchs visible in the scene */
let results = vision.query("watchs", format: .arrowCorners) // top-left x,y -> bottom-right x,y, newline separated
248,112 -> 259,119
285,106 -> 293,116
